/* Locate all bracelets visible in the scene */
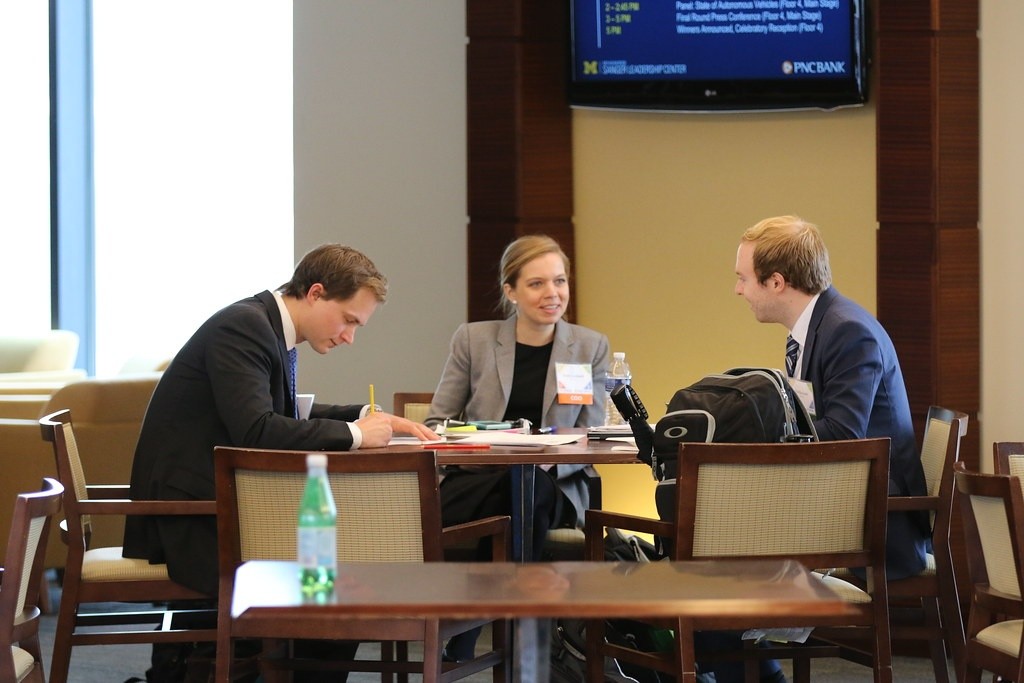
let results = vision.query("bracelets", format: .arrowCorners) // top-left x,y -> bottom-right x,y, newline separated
366,407 -> 382,415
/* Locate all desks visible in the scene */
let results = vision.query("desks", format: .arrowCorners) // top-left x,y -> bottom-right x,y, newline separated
355,428 -> 641,683
232,561 -> 872,683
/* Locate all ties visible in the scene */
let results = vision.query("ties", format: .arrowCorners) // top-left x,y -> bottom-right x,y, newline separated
785,333 -> 799,378
287,346 -> 299,421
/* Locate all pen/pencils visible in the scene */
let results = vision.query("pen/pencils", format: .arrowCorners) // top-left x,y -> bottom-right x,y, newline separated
369,383 -> 376,415
537,425 -> 558,434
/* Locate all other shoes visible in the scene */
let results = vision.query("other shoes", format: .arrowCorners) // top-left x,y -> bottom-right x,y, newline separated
764,669 -> 787,683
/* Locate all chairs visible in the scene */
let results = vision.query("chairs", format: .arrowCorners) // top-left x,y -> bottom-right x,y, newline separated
0,329 -> 1024,683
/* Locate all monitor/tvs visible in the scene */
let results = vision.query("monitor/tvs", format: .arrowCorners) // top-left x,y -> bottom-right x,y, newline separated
566,0 -> 871,112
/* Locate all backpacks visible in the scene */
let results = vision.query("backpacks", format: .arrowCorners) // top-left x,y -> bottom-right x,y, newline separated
651,367 -> 821,561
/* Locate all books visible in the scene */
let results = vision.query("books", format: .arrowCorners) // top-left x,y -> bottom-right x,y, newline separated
587,426 -> 636,440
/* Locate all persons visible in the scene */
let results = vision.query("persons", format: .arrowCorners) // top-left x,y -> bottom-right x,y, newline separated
424,234 -> 609,666
123,243 -> 440,683
698,216 -> 930,683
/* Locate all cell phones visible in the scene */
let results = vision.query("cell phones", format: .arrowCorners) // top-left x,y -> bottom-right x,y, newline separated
466,420 -> 511,431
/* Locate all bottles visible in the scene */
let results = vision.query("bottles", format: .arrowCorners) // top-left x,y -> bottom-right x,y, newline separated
294,453 -> 339,605
606,353 -> 632,428
